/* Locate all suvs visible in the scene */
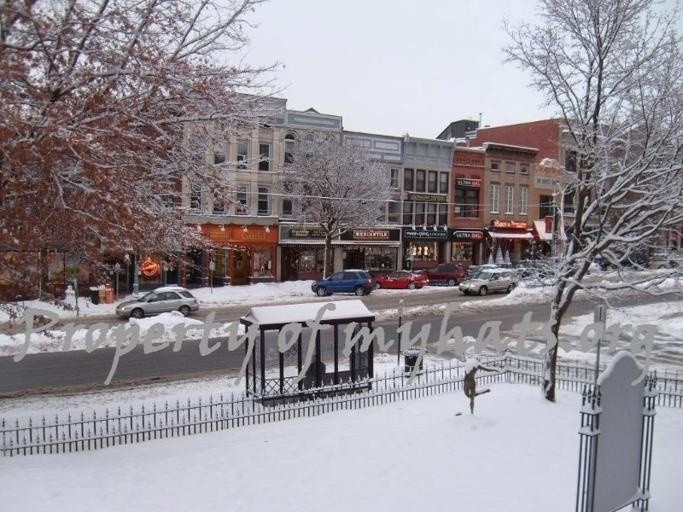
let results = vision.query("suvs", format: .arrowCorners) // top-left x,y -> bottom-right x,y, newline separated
311,269 -> 378,298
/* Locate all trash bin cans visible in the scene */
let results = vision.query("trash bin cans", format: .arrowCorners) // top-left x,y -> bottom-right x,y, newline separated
402,347 -> 428,378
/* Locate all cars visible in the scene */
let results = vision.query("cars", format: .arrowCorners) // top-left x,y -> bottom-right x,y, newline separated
414,241 -> 682,296
115,286 -> 200,320
373,268 -> 430,291
